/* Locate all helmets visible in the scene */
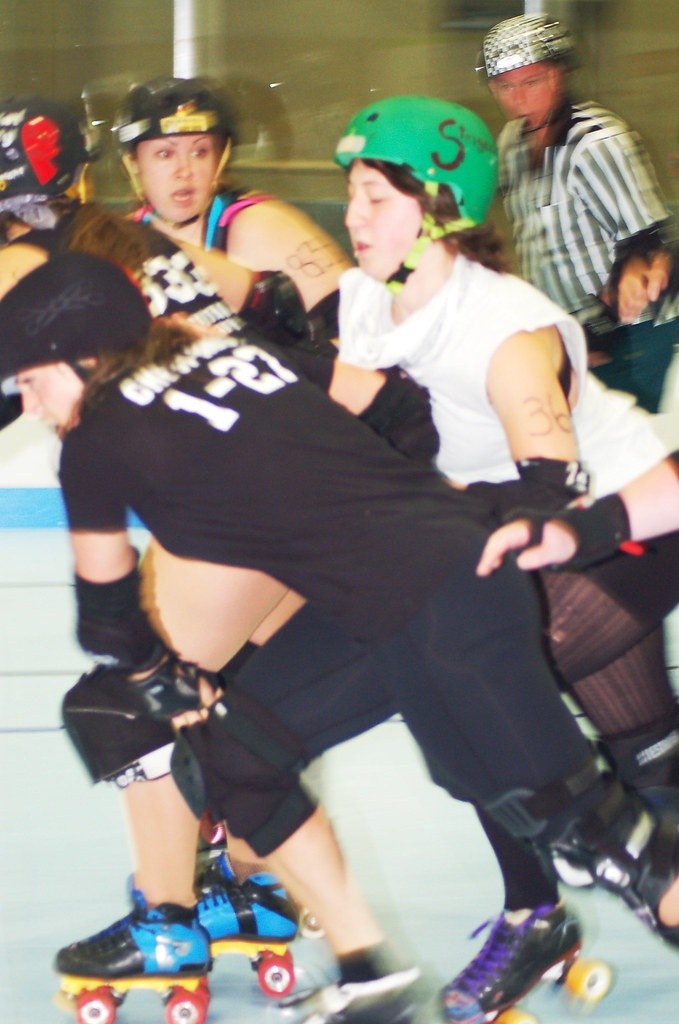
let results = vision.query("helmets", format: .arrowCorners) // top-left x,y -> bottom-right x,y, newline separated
0,253 -> 152,397
111,77 -> 232,229
0,97 -> 102,198
476,12 -> 576,135
333,94 -> 500,293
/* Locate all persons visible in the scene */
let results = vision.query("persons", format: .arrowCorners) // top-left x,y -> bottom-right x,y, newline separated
0,70 -> 440,1024
480,9 -> 673,321
472,232 -> 679,585
330,94 -> 679,1023
0,249 -> 678,1023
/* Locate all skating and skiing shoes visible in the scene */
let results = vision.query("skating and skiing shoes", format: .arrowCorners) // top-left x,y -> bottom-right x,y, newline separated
436,895 -> 611,1024
266,964 -> 431,1024
124,808 -> 324,939
546,702 -> 679,950
199,848 -> 299,997
50,871 -> 213,1024
